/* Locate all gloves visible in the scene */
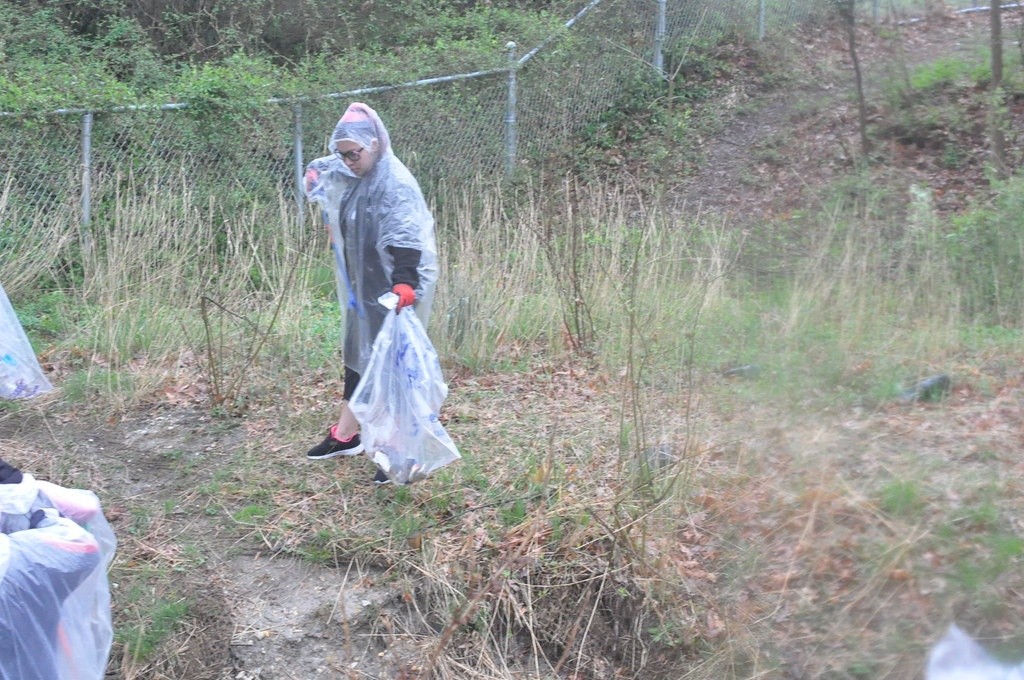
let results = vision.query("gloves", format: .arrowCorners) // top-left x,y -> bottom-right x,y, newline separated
392,283 -> 416,316
306,169 -> 319,194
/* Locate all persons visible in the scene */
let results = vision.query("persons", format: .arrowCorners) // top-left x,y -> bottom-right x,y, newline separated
0,458 -> 119,680
301,101 -> 441,487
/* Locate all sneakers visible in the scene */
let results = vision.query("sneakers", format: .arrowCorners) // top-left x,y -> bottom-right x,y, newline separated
306,426 -> 364,459
374,463 -> 402,483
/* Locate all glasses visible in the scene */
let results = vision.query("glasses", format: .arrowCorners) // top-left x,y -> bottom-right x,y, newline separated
333,147 -> 363,161
